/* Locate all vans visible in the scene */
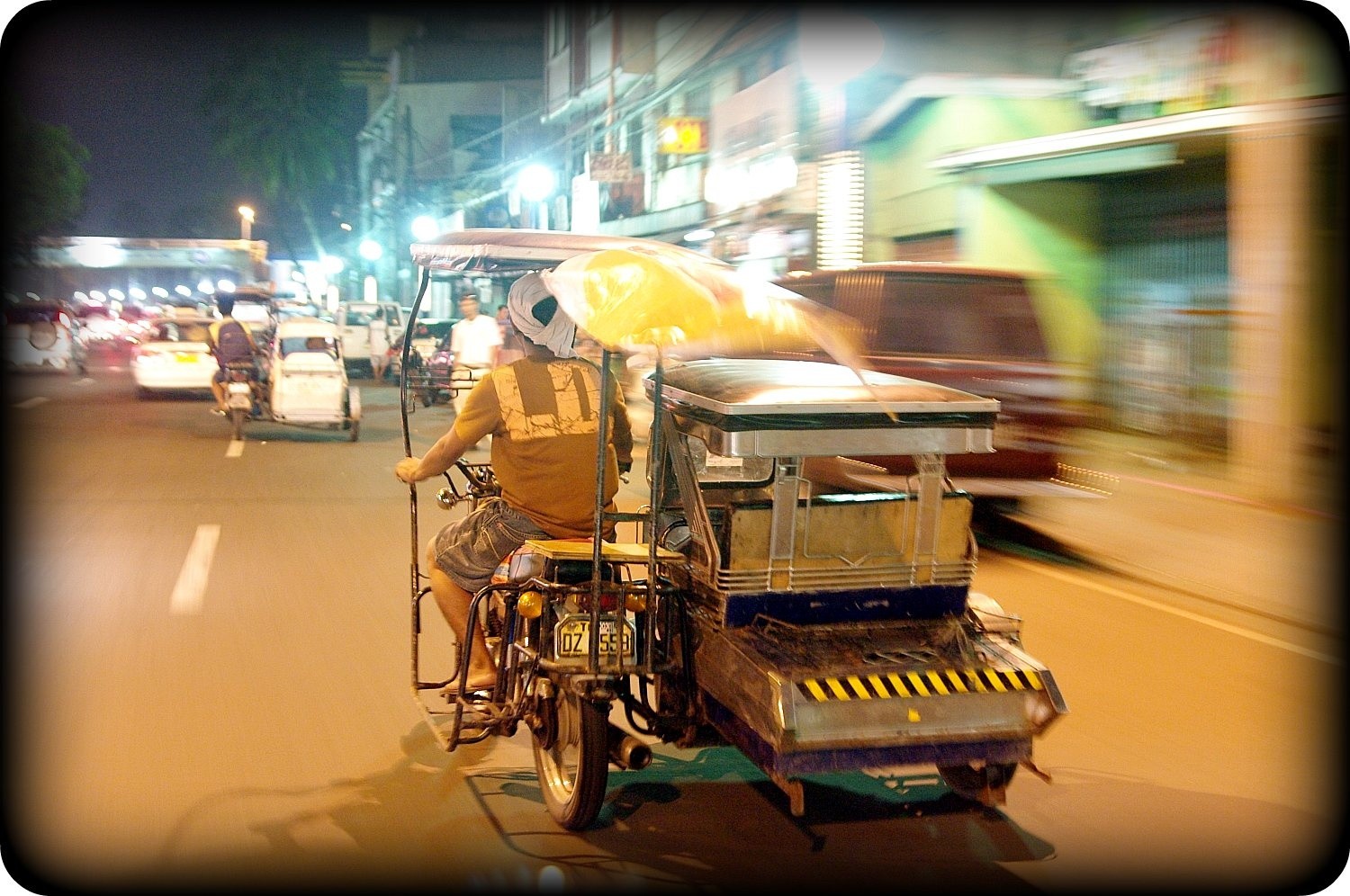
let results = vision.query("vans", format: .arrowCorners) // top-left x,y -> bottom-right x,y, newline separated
773,260 -> 1065,484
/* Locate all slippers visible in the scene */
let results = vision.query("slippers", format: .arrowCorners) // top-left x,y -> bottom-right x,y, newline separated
438,683 -> 495,697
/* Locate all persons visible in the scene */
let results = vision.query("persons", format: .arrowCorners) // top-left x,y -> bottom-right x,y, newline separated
366,310 -> 390,385
206,294 -> 262,416
601,180 -> 635,219
398,303 -> 515,393
394,272 -> 633,695
449,294 -> 504,452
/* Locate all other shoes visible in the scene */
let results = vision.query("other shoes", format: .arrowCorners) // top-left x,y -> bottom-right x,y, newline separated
210,406 -> 228,416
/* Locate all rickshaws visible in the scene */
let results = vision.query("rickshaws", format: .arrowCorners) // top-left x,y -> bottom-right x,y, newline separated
207,315 -> 364,444
398,226 -> 1071,832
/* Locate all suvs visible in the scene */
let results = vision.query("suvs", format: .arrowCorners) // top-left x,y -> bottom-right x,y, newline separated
0,300 -> 90,376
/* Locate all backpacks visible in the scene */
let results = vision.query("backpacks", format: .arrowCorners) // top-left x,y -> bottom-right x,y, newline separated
218,321 -> 251,362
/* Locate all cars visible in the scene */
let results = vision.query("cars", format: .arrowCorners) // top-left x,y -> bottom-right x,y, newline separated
125,291 -> 460,401
